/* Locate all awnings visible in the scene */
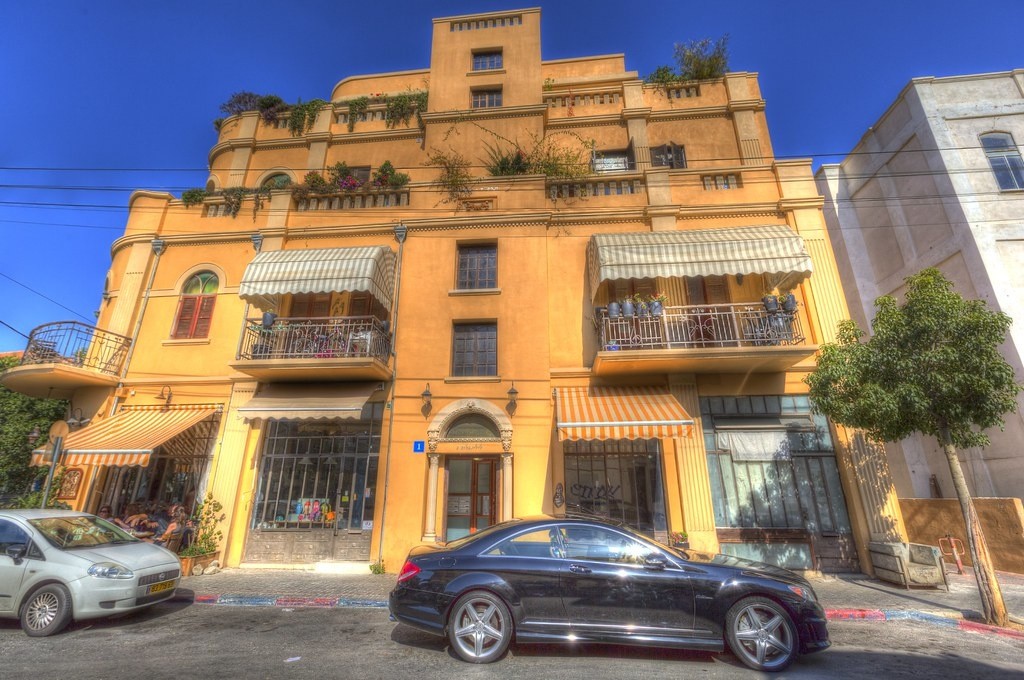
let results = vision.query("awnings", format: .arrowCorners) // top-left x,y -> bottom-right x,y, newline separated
238,245 -> 396,311
29,405 -> 221,468
238,381 -> 379,419
554,384 -> 695,440
585,224 -> 812,303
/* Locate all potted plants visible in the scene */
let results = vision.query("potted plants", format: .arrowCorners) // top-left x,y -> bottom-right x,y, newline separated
179,493 -> 228,578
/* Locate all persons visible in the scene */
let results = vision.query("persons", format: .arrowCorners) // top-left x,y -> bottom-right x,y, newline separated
98,503 -> 196,553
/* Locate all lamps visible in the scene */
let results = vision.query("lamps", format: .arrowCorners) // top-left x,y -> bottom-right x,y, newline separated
27,423 -> 49,445
421,383 -> 432,407
67,407 -> 89,428
506,380 -> 519,405
155,383 -> 172,402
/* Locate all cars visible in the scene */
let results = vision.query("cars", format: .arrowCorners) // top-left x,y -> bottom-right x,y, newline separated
387,510 -> 834,673
0,508 -> 182,637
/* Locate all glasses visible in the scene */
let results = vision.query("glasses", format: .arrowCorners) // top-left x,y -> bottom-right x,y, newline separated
99,510 -> 106,513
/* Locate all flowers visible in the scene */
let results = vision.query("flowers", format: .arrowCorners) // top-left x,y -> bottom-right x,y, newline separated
306,159 -> 410,191
759,291 -> 793,302
623,290 -> 669,303
666,532 -> 688,544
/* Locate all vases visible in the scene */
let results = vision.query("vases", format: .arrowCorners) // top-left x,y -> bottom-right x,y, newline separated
622,302 -> 634,318
761,294 -> 778,313
782,294 -> 797,310
249,310 -> 278,360
607,302 -> 620,319
673,541 -> 689,548
634,302 -> 649,319
649,301 -> 662,317
